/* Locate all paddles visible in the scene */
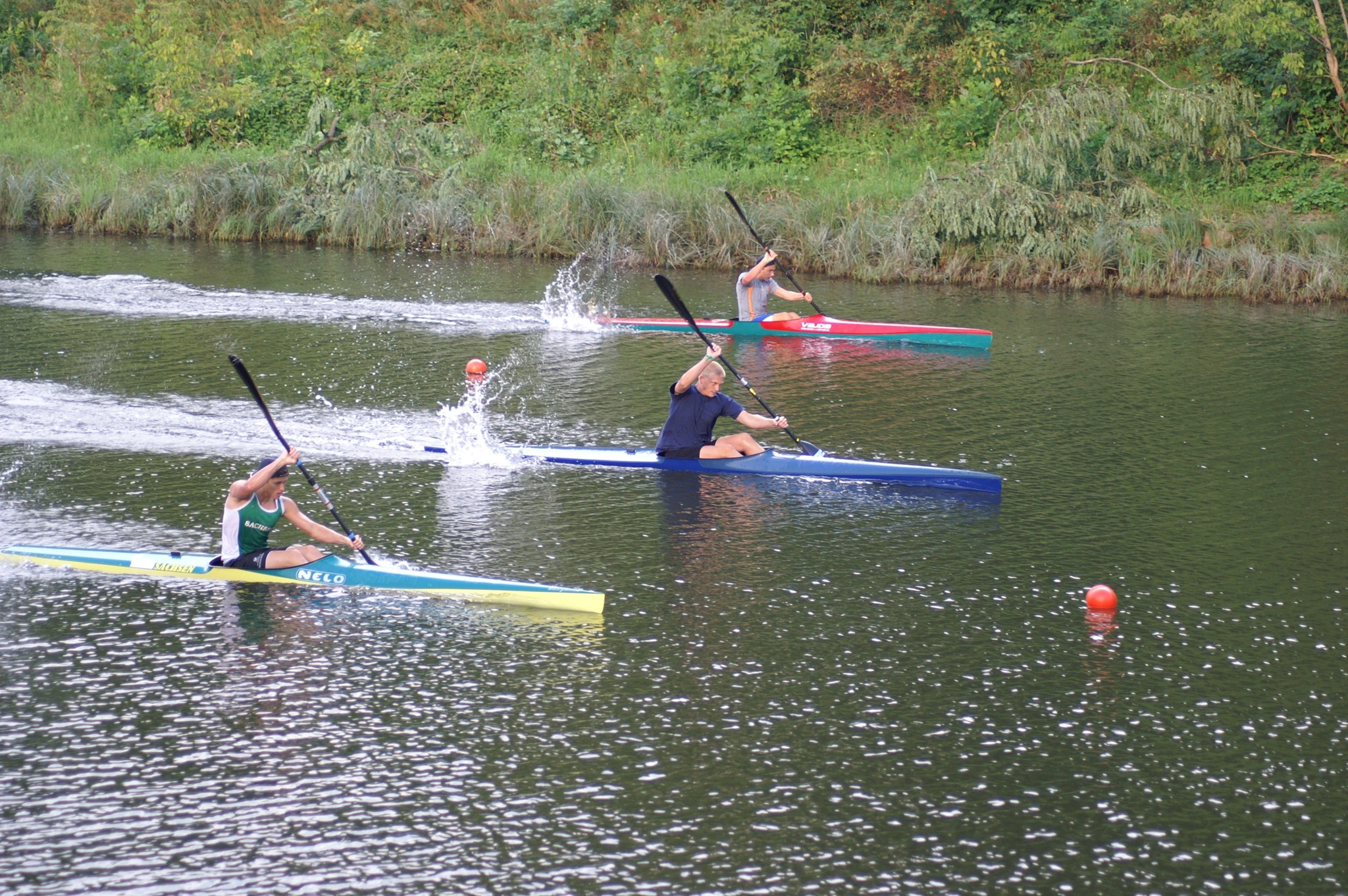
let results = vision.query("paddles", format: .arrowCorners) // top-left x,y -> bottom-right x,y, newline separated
724,190 -> 828,315
654,274 -> 824,455
226,353 -> 377,566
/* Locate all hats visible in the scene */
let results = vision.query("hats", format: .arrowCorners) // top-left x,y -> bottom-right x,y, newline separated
250,456 -> 289,478
756,255 -> 775,267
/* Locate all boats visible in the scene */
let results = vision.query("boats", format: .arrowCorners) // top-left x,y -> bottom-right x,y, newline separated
0,543 -> 605,613
595,314 -> 992,349
424,443 -> 1003,493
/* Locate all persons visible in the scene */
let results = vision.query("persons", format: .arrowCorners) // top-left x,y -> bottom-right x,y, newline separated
736,248 -> 812,322
656,342 -> 788,458
221,445 -> 364,568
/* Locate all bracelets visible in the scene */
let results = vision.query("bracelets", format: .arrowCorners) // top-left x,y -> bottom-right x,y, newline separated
704,354 -> 714,361
